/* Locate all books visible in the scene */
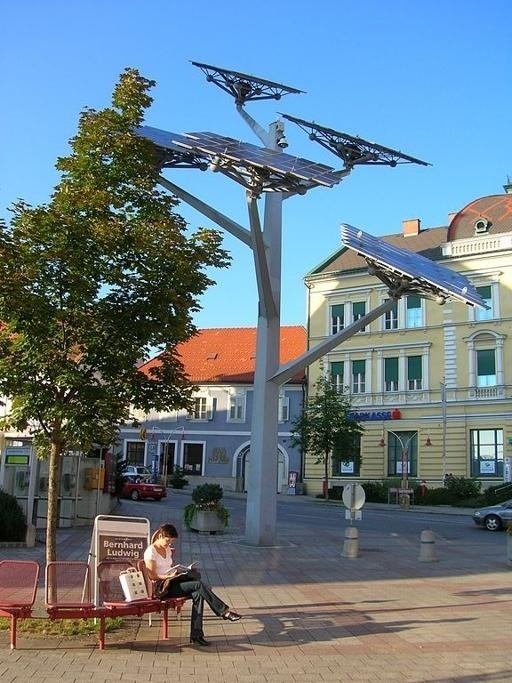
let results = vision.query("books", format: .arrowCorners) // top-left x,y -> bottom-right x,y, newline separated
164,560 -> 200,572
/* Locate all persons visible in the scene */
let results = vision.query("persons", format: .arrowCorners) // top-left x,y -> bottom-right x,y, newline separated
144,523 -> 242,645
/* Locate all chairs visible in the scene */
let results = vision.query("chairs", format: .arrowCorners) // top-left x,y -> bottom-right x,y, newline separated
0,560 -> 190,651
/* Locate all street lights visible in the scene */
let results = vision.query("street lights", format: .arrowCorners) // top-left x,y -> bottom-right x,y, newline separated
379,428 -> 432,489
150,425 -> 186,486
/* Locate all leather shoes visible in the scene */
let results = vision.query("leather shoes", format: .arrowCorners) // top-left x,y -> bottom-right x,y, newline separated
189,636 -> 210,646
221,610 -> 242,622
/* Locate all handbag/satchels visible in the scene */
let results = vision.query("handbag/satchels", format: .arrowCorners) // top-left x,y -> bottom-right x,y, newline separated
116,565 -> 150,601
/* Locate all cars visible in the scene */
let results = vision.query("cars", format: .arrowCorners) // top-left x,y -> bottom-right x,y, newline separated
118,463 -> 167,501
472,497 -> 512,531
